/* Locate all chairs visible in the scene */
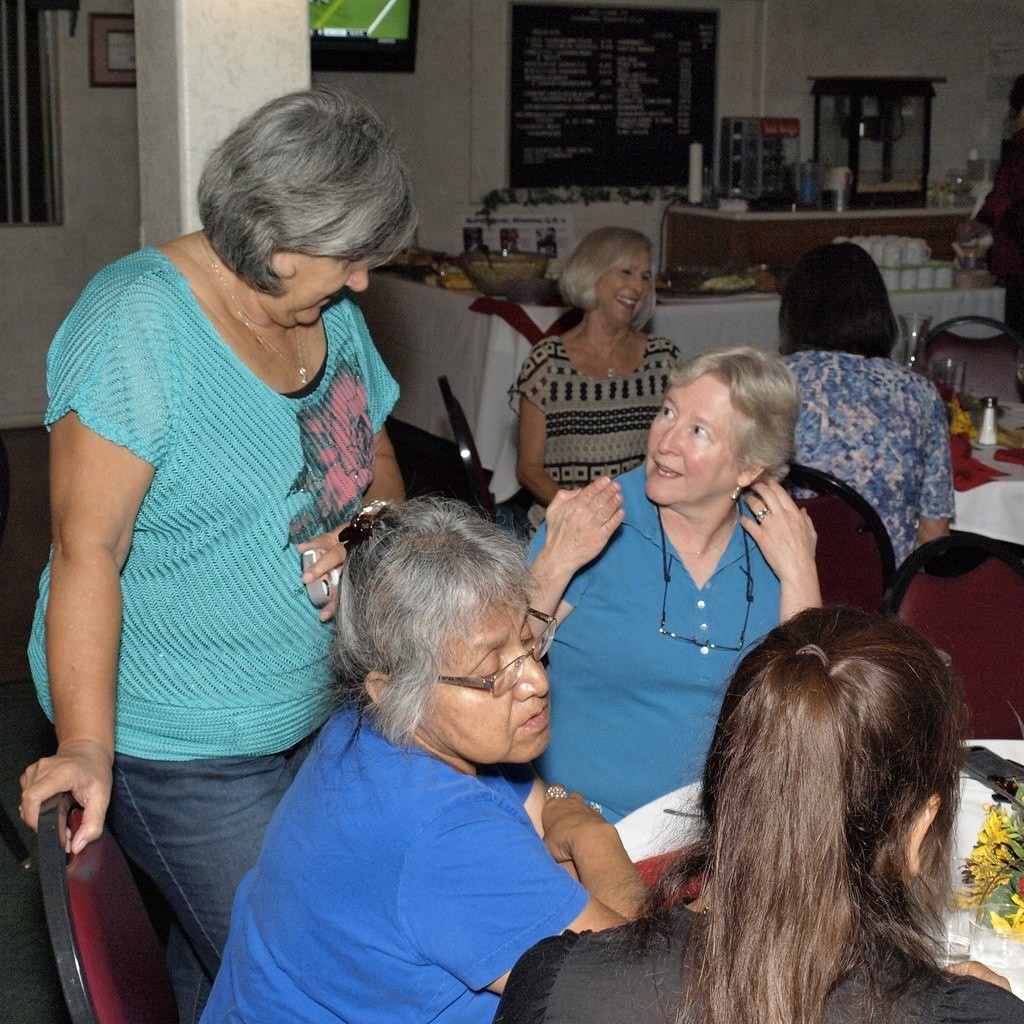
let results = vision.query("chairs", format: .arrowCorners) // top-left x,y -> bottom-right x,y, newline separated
883,533 -> 1024,741
437,373 -> 497,525
37,791 -> 185,1024
922,315 -> 1024,404
781,462 -> 895,614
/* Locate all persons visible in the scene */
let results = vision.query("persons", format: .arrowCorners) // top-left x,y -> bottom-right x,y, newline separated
494,604 -> 1024,1024
197,497 -> 649,1023
512,227 -> 682,536
19,90 -> 419,1023
524,346 -> 823,822
964,72 -> 1024,352
778,243 -> 955,570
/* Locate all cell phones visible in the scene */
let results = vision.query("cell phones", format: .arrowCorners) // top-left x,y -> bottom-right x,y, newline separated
961,746 -> 1024,793
301,548 -> 335,606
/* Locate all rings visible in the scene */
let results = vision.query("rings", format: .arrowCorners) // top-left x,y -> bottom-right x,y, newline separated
588,801 -> 602,816
756,508 -> 770,522
545,786 -> 567,800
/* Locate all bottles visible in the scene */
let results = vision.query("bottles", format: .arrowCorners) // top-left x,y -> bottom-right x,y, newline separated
978,397 -> 1000,445
798,158 -> 819,205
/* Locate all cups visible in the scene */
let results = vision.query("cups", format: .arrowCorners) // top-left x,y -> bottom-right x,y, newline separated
921,859 -> 992,965
830,231 -> 954,294
967,901 -> 1024,986
825,165 -> 852,200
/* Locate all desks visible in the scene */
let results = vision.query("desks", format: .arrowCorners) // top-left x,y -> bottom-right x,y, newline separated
351,265 -> 1006,508
615,741 -> 1024,1003
947,396 -> 1024,547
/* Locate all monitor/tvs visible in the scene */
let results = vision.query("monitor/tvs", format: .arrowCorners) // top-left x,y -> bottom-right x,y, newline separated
307,0 -> 419,74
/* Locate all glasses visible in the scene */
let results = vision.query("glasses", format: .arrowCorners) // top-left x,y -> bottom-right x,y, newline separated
432,608 -> 559,699
659,556 -> 761,653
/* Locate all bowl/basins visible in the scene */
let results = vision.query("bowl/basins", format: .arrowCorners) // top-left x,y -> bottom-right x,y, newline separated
464,250 -> 549,294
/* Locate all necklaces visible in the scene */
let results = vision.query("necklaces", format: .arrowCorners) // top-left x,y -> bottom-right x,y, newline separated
581,334 -> 635,378
658,502 -> 739,556
200,229 -> 309,387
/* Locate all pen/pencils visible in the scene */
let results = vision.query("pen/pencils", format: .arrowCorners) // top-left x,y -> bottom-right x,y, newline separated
664,809 -> 703,818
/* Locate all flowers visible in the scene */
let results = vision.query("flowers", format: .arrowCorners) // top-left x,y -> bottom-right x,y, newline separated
945,777 -> 1024,943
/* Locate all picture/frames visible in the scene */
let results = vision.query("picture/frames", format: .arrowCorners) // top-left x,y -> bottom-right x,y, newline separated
86,12 -> 136,88
